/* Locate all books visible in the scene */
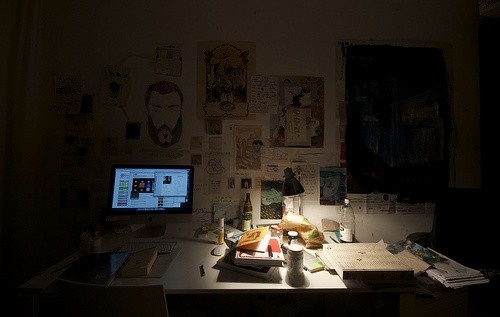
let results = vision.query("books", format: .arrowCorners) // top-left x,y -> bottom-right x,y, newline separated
114,248 -> 158,277
217,248 -> 276,278
55,253 -> 130,286
236,226 -> 271,252
322,242 -> 415,280
316,251 -> 335,271
234,236 -> 285,267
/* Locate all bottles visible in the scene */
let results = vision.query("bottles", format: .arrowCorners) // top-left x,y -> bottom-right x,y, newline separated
242,192 -> 252,231
340,198 -> 356,243
287,230 -> 299,245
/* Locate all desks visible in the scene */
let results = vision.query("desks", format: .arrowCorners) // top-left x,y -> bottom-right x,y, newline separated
50,225 -> 348,292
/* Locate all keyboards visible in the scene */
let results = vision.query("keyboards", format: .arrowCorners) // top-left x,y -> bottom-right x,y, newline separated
115,239 -> 178,254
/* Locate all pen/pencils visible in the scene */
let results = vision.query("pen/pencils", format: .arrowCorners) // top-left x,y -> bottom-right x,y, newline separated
315,253 -> 329,271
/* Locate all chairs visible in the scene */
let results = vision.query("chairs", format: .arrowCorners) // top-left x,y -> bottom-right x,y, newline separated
406,188 -> 500,267
55,278 -> 169,317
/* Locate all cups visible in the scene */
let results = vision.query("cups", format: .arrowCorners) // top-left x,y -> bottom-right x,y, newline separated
80,237 -> 101,254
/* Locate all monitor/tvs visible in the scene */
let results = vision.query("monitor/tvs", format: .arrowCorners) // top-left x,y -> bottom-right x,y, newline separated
107,164 -> 195,224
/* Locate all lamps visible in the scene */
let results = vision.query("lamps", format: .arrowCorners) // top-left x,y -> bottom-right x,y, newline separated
283,166 -> 305,214
